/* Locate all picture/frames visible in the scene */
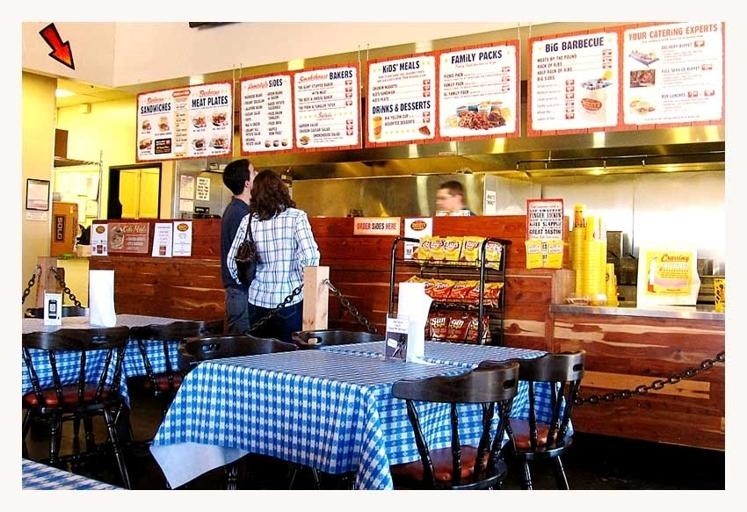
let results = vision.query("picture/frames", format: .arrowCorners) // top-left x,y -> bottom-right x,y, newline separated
26,178 -> 50,211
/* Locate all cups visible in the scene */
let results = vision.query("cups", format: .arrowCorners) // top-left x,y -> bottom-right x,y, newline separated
373,118 -> 381,139
713,278 -> 723,311
176,224 -> 190,243
565,204 -> 619,307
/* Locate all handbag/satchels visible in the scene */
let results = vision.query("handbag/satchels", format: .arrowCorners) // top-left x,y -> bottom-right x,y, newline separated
234,211 -> 261,291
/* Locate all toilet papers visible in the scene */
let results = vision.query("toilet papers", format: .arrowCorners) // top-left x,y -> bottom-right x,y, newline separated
397,283 -> 432,357
89,269 -> 117,328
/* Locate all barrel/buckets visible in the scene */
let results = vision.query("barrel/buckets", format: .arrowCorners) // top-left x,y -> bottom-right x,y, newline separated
50,202 -> 79,256
293,328 -> 386,351
177,332 -> 294,383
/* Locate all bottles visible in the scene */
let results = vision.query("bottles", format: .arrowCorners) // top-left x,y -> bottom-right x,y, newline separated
347,209 -> 364,217
94,226 -> 105,242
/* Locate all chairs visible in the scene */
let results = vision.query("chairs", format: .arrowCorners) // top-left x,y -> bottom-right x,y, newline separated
178,335 -> 298,378
131,320 -> 223,417
506,351 -> 585,489
292,331 -> 385,350
25,306 -> 89,319
22,326 -> 135,488
390,362 -> 519,489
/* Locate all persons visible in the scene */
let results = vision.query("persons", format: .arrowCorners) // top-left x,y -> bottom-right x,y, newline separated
221,159 -> 259,334
226,170 -> 320,342
434,181 -> 477,216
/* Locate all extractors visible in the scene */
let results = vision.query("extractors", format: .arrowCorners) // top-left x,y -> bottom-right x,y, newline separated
230,127 -> 724,181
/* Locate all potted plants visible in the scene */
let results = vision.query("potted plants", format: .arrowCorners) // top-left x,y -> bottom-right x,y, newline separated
76,225 -> 91,257
